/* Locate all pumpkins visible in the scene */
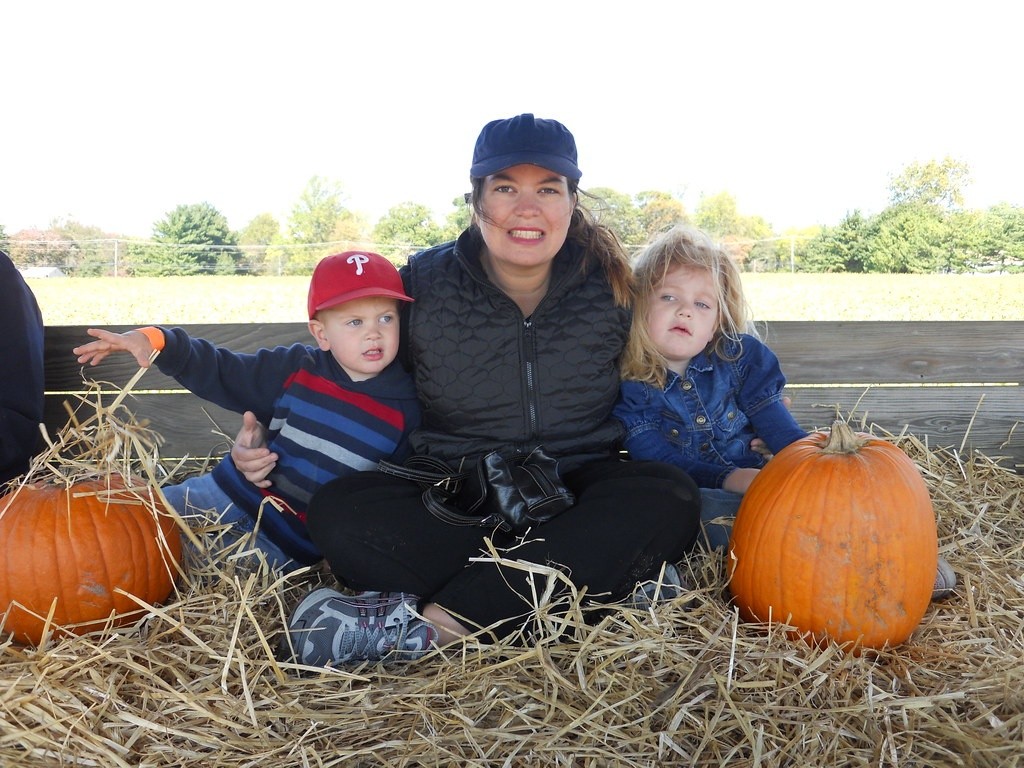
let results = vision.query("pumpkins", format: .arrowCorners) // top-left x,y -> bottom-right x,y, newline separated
724,418 -> 937,659
0,465 -> 181,647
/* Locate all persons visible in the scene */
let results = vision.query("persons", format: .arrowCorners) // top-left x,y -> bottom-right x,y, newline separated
73,251 -> 414,593
0,251 -> 46,498
612,228 -> 956,612
228,113 -> 790,679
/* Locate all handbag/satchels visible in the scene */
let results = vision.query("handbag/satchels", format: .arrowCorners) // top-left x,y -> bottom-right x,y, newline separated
375,440 -> 576,532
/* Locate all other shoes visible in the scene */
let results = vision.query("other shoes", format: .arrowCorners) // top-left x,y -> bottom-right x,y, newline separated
930,556 -> 956,600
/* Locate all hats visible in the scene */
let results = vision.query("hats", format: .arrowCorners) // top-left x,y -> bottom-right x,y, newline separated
469,113 -> 582,180
307,250 -> 415,320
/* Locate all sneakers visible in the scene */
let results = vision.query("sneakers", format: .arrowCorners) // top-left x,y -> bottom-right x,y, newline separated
278,587 -> 440,669
626,562 -> 686,614
